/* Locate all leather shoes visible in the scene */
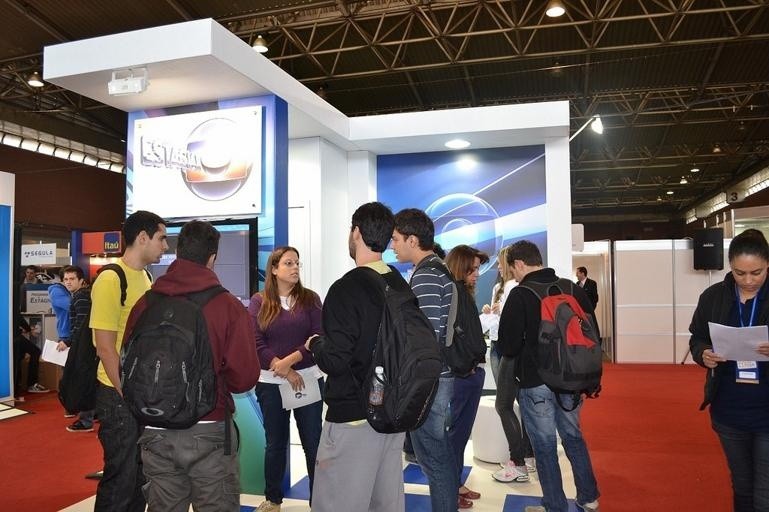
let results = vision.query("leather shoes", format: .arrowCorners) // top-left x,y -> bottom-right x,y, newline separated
458,489 -> 480,508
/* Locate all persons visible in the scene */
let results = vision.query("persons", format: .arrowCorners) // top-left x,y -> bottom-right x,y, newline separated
56,266 -> 103,431
47,265 -> 77,418
482,245 -> 536,482
497,241 -> 600,508
392,208 -> 487,508
15,295 -> 52,393
23,263 -> 39,284
575,267 -> 598,311
688,229 -> 767,512
244,242 -> 326,510
305,202 -> 413,509
85,208 -> 168,511
115,217 -> 257,512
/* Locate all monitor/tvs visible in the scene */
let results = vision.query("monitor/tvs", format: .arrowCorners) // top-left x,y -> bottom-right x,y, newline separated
144,218 -> 259,311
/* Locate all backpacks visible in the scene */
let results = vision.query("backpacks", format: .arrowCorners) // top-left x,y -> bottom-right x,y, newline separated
516,278 -> 602,393
119,286 -> 229,430
409,260 -> 487,379
57,264 -> 151,414
322,265 -> 442,434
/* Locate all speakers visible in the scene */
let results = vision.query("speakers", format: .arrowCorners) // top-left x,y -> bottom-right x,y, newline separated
692,227 -> 725,270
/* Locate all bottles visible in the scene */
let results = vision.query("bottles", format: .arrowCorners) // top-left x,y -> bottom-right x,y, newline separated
368,366 -> 384,407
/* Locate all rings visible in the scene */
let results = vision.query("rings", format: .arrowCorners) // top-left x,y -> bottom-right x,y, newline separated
704,354 -> 710,360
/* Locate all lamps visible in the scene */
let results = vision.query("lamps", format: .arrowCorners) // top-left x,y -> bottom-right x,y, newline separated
27,72 -> 45,88
545,0 -> 566,18
252,35 -> 269,53
657,143 -> 721,203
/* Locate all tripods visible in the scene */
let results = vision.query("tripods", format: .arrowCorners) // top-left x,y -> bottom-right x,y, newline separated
680,271 -> 724,365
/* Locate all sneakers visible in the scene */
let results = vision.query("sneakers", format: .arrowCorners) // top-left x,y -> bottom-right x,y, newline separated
492,457 -> 536,483
28,382 -> 49,392
253,500 -> 280,512
575,496 -> 600,512
525,505 -> 546,512
67,419 -> 94,432
